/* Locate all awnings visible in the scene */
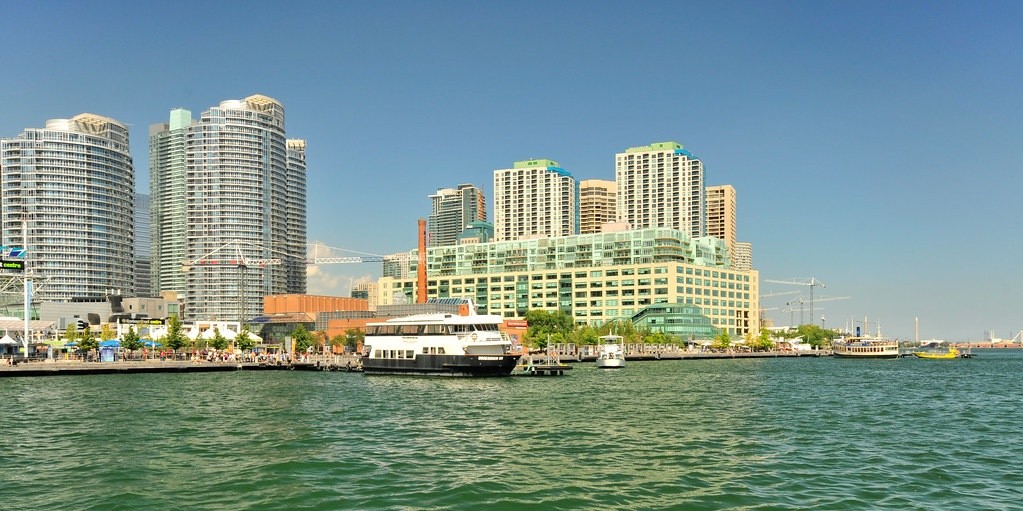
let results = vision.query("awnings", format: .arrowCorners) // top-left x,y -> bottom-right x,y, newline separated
238,330 -> 263,344
145,326 -> 173,343
0,331 -> 18,344
218,327 -> 237,343
181,327 -> 203,343
198,329 -> 219,345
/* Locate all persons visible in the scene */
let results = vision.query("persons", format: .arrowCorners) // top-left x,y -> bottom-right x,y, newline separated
3,346 -> 370,368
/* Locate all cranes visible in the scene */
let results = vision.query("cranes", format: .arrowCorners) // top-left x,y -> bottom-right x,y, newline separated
179,238 -> 392,330
759,274 -> 852,329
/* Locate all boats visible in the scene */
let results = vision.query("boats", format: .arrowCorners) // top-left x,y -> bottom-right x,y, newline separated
831,337 -> 899,357
360,311 -> 522,378
596,334 -> 627,369
915,346 -> 959,360
